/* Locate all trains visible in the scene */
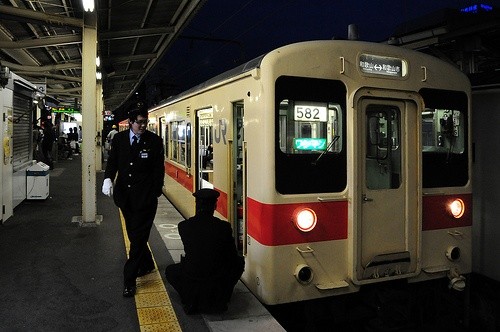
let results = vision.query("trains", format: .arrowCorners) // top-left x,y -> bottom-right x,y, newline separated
119,24 -> 473,306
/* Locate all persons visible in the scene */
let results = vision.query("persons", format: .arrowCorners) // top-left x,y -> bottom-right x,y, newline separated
102,99 -> 165,298
166,188 -> 245,315
107,124 -> 118,149
67,126 -> 82,161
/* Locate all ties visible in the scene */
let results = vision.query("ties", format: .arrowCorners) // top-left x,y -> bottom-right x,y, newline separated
132,136 -> 138,146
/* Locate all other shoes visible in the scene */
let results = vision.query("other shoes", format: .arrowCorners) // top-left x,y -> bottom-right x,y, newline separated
123,288 -> 136,296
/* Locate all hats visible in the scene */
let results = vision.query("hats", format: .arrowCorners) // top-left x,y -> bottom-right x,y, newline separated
192,189 -> 220,202
112,125 -> 116,128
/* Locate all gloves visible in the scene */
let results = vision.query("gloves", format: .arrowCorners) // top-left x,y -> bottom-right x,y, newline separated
102,179 -> 113,196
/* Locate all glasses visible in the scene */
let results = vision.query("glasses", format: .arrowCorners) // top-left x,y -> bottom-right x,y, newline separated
134,119 -> 149,125
